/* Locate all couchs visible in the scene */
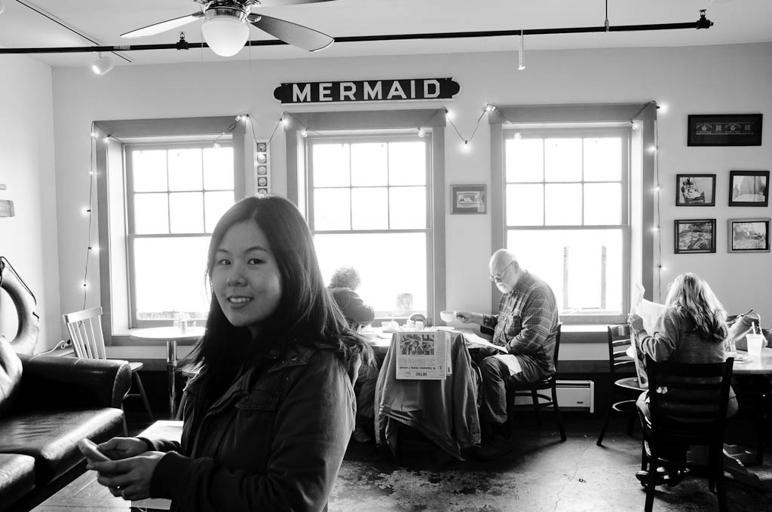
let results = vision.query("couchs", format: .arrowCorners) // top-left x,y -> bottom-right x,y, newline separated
0,347 -> 133,512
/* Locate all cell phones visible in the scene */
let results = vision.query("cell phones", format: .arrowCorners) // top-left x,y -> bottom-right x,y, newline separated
76,438 -> 110,463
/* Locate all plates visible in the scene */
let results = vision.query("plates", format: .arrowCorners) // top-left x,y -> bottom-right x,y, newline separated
435,325 -> 454,330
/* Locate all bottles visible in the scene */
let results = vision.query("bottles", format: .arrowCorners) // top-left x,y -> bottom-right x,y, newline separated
180,320 -> 187,329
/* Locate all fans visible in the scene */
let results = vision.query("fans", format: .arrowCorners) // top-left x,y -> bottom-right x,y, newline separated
118,1 -> 339,56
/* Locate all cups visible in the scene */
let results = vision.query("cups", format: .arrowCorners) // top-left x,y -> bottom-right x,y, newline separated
440,310 -> 458,323
744,334 -> 763,358
400,319 -> 425,331
723,338 -> 738,358
382,321 -> 398,331
398,292 -> 412,314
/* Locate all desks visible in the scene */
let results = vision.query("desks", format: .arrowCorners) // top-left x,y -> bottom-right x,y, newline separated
25,416 -> 193,512
125,321 -> 213,426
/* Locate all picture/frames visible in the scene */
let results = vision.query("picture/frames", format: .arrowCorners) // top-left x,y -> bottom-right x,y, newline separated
671,110 -> 771,257
447,183 -> 490,216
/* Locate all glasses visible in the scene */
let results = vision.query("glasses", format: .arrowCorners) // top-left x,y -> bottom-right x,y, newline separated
490,263 -> 512,282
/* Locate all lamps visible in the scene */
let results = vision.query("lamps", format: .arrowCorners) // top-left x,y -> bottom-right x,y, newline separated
200,5 -> 252,60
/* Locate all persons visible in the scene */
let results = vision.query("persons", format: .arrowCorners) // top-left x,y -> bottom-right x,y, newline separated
325,260 -> 384,461
455,242 -> 564,463
82,194 -> 363,512
623,266 -> 740,485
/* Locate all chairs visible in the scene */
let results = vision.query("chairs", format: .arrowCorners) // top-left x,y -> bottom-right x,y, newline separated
379,328 -> 467,474
61,305 -> 156,436
502,321 -> 572,446
640,354 -> 736,512
597,323 -> 643,446
